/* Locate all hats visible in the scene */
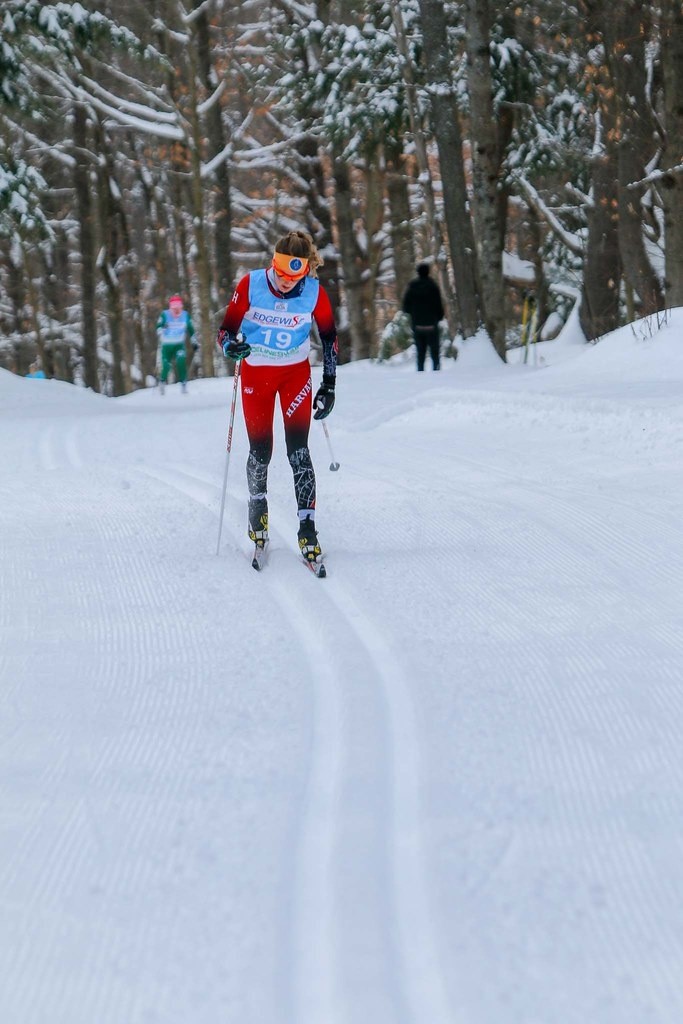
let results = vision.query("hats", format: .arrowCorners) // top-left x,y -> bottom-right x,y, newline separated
168,297 -> 184,309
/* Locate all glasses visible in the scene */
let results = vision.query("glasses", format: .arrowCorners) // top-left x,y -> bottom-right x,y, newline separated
273,260 -> 309,281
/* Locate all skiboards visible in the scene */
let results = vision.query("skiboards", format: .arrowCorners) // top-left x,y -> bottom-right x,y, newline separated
251,537 -> 327,579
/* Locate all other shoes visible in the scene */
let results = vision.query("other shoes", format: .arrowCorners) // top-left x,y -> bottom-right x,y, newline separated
180,381 -> 188,394
159,383 -> 165,394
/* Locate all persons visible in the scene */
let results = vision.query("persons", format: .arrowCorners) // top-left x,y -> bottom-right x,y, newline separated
25,363 -> 45,379
218,230 -> 338,563
155,295 -> 202,395
401,263 -> 445,371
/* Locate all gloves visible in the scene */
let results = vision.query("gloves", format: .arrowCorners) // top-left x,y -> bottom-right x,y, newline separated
313,376 -> 336,420
221,332 -> 251,361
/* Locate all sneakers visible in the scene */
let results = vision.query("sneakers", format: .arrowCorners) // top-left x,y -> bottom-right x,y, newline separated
247,495 -> 270,571
297,514 -> 326,577
